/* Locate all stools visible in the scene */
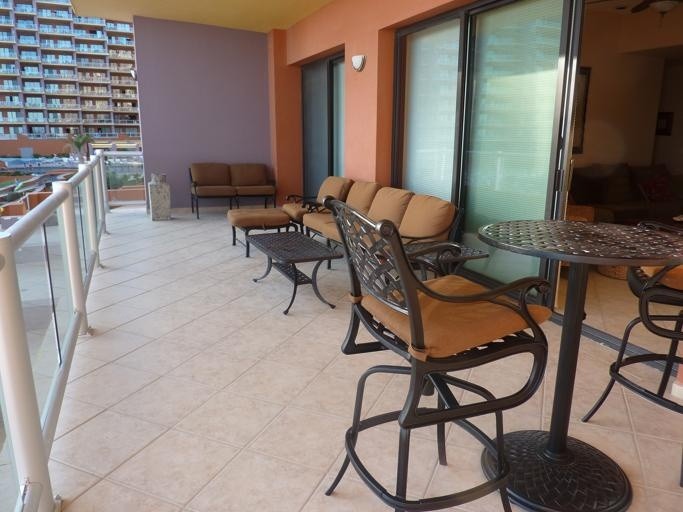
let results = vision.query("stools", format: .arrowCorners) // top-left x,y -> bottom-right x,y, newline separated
225,207 -> 299,259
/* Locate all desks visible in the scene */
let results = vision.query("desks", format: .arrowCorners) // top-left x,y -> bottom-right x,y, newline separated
402,239 -> 491,280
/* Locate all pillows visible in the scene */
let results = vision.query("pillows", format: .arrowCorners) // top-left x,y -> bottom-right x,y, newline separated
571,162 -> 638,205
635,173 -> 675,205
627,161 -> 673,177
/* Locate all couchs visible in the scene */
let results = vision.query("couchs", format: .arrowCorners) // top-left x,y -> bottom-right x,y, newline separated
187,161 -> 278,221
302,178 -> 468,285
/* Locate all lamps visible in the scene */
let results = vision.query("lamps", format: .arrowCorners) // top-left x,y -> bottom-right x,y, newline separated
350,53 -> 366,73
649,0 -> 679,16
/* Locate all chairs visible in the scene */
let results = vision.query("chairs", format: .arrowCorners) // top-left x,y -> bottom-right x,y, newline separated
578,217 -> 682,491
280,175 -> 356,235
322,192 -> 554,512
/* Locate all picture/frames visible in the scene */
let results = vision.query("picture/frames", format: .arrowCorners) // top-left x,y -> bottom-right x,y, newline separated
572,66 -> 593,156
